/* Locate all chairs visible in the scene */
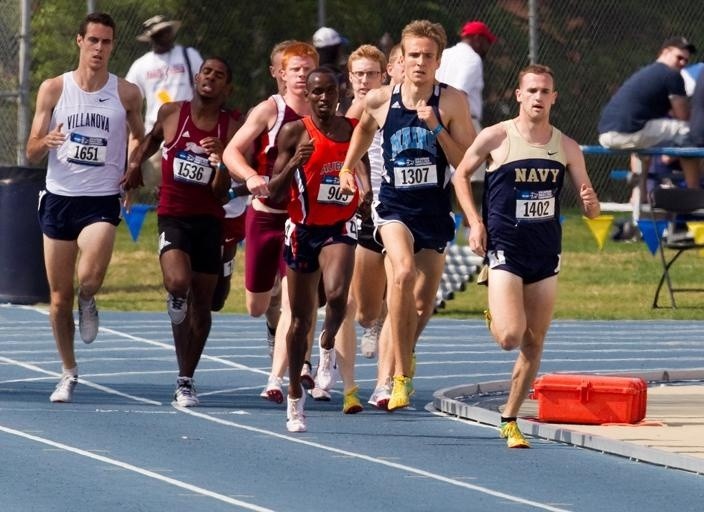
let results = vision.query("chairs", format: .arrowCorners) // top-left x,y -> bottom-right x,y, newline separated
166,286 -> 191,326
173,376 -> 199,409
259,272 -> 417,433
77,288 -> 99,344
48,361 -> 79,404
499,416 -> 531,448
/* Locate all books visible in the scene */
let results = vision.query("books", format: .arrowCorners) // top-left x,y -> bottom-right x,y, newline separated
531,372 -> 647,429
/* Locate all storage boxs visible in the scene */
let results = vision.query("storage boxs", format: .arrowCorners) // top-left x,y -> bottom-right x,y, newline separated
575,138 -> 704,232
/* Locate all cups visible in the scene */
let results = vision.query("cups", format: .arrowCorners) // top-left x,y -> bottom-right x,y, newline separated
350,69 -> 383,81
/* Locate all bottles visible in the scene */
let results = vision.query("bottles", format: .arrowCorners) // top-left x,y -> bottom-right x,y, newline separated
310,25 -> 350,50
459,19 -> 497,45
134,12 -> 183,42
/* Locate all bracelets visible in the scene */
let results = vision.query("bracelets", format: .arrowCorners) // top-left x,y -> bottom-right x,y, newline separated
433,124 -> 442,134
339,169 -> 353,176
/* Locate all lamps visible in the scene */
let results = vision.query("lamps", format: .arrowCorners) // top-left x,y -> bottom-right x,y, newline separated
644,186 -> 704,313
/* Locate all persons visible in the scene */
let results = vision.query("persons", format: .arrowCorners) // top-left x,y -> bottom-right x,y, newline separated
119,57 -> 252,407
315,27 -> 407,409
449,62 -> 601,449
596,34 -> 701,250
436,20 -> 498,240
338,20 -> 476,410
25,11 -> 147,405
221,41 -> 319,403
268,68 -> 375,433
129,18 -> 206,194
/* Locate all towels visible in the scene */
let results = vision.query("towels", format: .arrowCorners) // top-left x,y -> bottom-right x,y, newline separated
1,166 -> 50,303
